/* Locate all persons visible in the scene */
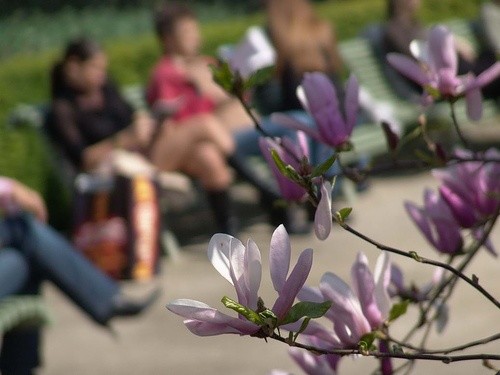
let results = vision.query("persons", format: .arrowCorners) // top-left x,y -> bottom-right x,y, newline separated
258,0 -> 371,203
382,0 -> 500,109
41,35 -> 158,283
0,176 -> 164,375
145,1 -> 310,238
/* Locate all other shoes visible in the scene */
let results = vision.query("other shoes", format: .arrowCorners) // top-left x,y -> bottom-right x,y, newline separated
268,206 -> 311,234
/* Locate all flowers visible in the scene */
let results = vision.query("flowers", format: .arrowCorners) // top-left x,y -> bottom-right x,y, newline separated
159,24 -> 500,372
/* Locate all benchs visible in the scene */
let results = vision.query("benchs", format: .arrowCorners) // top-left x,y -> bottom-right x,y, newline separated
335,16 -> 499,169
16,86 -> 263,265
2,297 -> 51,375
241,65 -> 390,206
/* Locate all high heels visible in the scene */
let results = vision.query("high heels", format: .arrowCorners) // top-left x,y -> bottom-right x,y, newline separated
100,286 -> 162,332
